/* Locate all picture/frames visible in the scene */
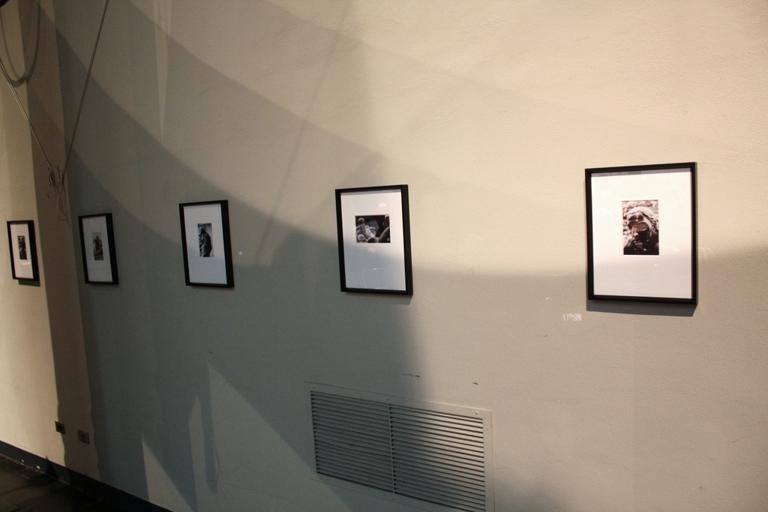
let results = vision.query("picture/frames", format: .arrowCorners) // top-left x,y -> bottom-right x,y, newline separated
584,162 -> 698,306
77,213 -> 119,285
178,200 -> 234,288
334,184 -> 414,296
6,219 -> 39,281
